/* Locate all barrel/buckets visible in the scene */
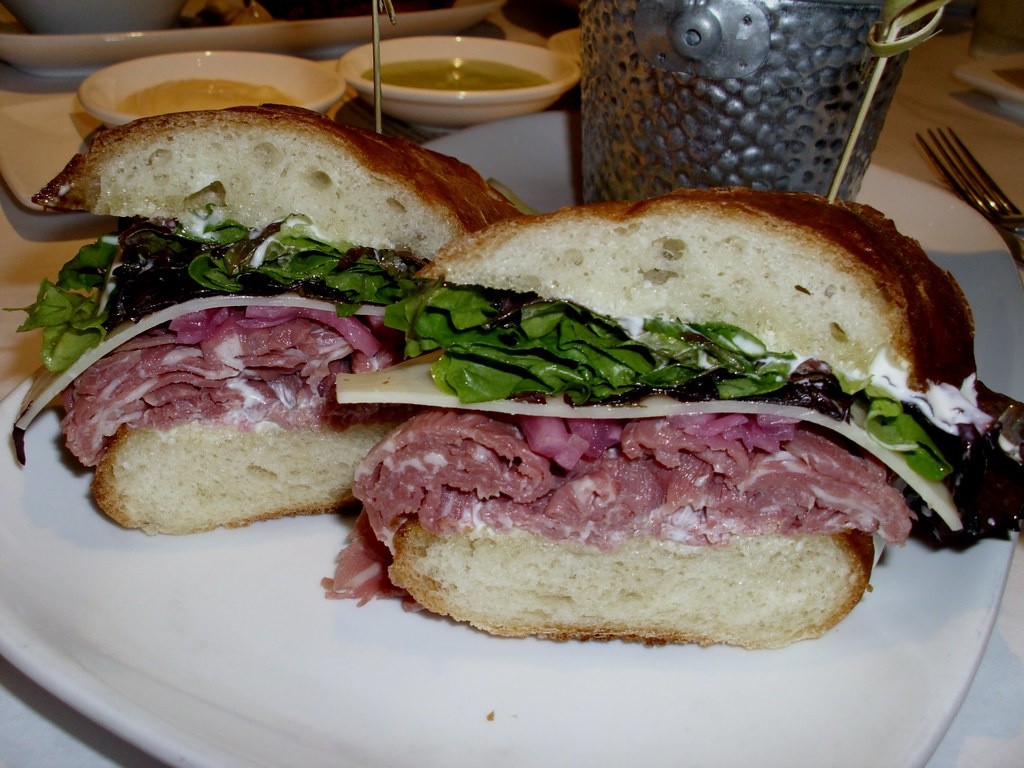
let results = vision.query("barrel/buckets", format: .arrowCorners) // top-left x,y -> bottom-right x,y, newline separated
580,0 -> 924,208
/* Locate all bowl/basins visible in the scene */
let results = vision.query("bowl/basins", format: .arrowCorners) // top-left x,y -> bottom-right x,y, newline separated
78,50 -> 347,128
337,35 -> 579,137
1,0 -> 185,33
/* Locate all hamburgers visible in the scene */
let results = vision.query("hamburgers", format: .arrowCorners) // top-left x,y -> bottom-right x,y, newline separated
320,188 -> 1024,649
2,104 -> 524,534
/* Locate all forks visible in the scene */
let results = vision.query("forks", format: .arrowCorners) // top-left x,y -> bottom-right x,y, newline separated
914,126 -> 1024,263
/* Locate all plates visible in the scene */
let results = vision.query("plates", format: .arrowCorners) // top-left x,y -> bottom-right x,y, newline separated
952,53 -> 1024,122
0,0 -> 505,80
0,110 -> 1024,768
